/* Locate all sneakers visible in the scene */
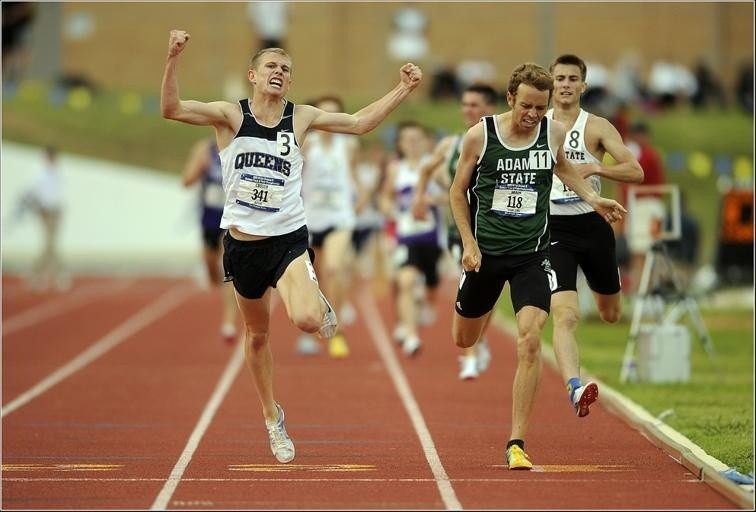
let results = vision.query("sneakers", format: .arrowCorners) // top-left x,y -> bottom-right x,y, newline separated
572,382 -> 598,417
505,443 -> 533,470
265,401 -> 296,464
456,334 -> 491,380
318,289 -> 338,340
394,307 -> 435,356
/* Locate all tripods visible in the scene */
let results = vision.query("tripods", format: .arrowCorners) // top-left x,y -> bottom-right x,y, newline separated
619,219 -> 718,386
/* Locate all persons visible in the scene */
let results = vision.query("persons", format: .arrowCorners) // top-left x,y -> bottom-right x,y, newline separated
430,84 -> 497,378
298,93 -> 361,356
542,51 -> 646,419
11,131 -> 77,300
379,120 -> 441,360
354,139 -> 388,277
579,86 -> 666,302
182,137 -> 238,341
158,21 -> 426,468
448,59 -> 629,472
